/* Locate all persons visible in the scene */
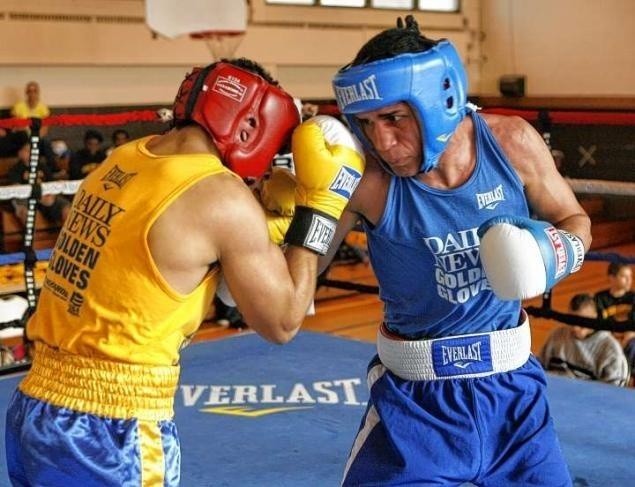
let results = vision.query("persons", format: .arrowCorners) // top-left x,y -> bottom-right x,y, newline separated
538,260 -> 635,387
0,82 -> 131,230
213,26 -> 594,487
5,57 -> 367,487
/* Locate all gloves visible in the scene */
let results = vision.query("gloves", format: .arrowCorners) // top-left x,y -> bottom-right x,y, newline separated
215,168 -> 297,325
283,116 -> 366,255
477,215 -> 584,301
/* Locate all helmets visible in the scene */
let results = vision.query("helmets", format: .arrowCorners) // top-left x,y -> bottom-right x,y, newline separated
332,37 -> 467,180
173,62 -> 301,181
